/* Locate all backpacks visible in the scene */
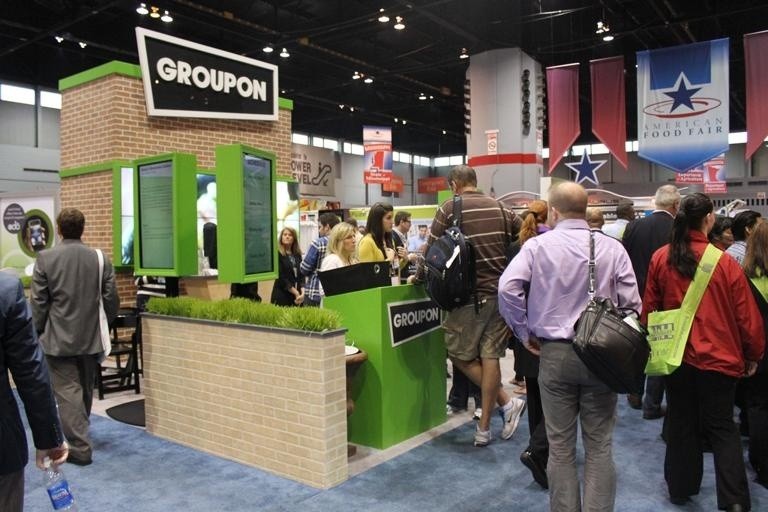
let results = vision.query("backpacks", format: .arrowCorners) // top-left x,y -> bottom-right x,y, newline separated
424,193 -> 484,315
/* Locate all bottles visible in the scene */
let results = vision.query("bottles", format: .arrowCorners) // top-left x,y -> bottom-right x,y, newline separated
40,458 -> 75,511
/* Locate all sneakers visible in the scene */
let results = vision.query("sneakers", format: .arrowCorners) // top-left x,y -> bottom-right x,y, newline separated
446,378 -> 528,441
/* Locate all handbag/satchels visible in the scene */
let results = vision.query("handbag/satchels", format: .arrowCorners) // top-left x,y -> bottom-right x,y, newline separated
573,228 -> 652,395
95,248 -> 112,358
643,242 -> 724,376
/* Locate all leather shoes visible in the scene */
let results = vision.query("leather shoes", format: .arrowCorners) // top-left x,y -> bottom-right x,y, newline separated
643,405 -> 667,420
66,455 -> 92,467
521,449 -> 551,489
628,393 -> 643,409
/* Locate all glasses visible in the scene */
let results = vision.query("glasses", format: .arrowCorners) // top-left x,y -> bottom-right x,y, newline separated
406,221 -> 411,224
338,234 -> 356,240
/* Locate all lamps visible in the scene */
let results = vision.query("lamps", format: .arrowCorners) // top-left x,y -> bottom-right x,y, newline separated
460,48 -> 469,59
379,8 -> 405,30
136,3 -> 173,23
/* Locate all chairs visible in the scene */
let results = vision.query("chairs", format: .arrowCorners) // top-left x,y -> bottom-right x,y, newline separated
97,312 -> 141,400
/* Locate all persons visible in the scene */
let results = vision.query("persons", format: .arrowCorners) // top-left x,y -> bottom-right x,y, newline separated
405,166 -> 529,445
637,194 -> 766,511
0,266 -> 72,511
27,207 -> 121,465
496,182 -> 648,510
513,186 -> 766,490
274,199 -> 428,308
